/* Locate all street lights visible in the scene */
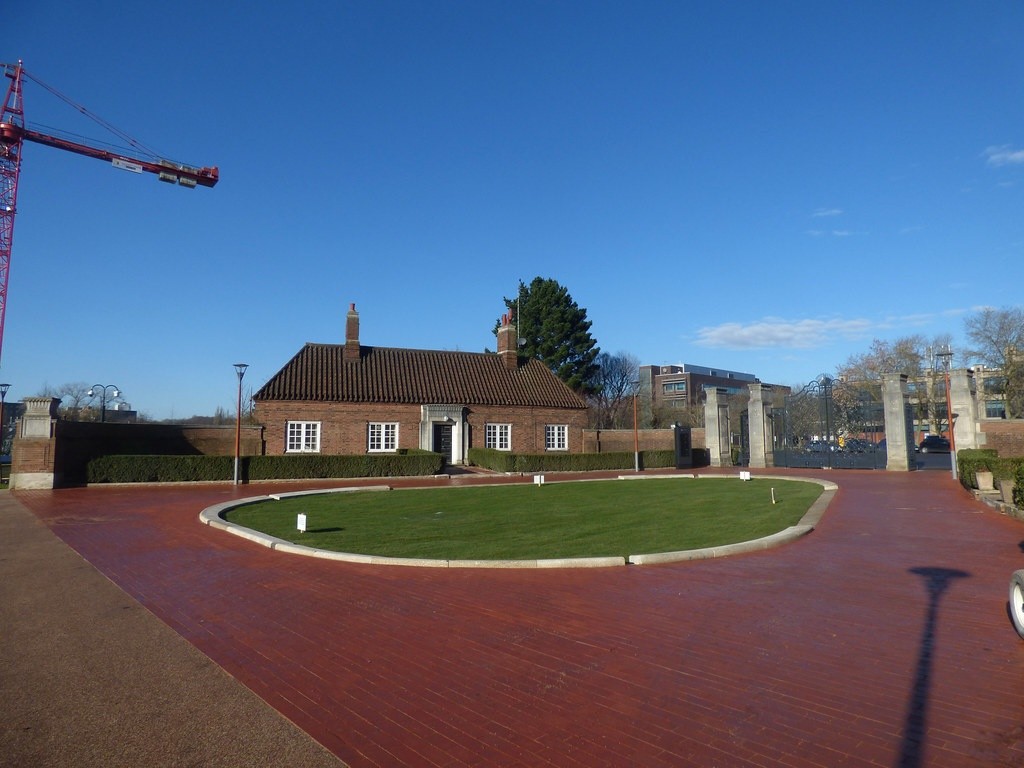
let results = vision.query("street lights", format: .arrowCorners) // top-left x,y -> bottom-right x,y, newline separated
936,346 -> 958,479
232,364 -> 249,485
87,384 -> 120,422
628,380 -> 641,472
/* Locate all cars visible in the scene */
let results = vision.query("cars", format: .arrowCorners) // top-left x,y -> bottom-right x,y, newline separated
803,438 -> 919,456
919,435 -> 950,454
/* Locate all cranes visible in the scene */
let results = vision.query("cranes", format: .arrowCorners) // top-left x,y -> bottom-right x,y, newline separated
0,56 -> 221,360
